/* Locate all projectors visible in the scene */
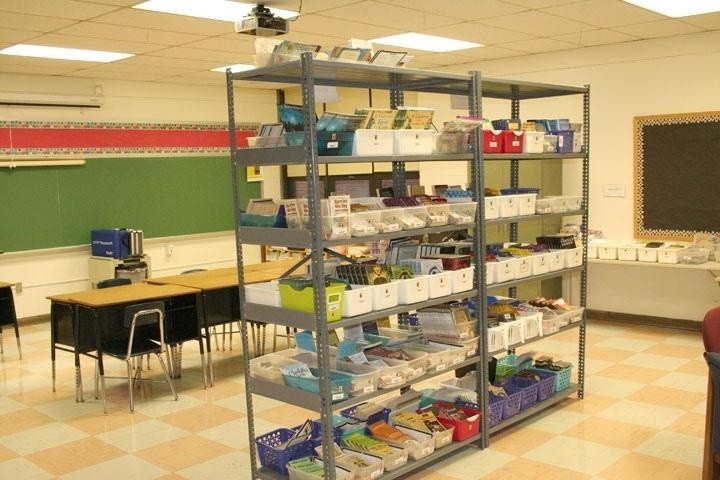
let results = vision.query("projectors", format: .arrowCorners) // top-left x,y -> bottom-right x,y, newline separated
235,16 -> 289,38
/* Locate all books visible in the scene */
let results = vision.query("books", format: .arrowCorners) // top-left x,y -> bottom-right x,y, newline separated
246,102 -> 583,478
266,40 -> 408,67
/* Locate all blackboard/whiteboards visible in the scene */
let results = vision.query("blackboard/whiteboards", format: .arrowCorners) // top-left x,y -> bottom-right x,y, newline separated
0,153 -> 264,256
633,111 -> 720,243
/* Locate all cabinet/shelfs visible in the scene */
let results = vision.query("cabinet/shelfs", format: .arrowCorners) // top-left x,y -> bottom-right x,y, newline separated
225,52 -> 591,480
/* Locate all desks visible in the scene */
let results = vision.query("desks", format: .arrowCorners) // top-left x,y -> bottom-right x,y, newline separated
0,281 -> 23,359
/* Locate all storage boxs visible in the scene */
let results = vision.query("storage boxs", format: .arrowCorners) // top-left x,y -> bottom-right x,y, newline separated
588,239 -> 692,263
